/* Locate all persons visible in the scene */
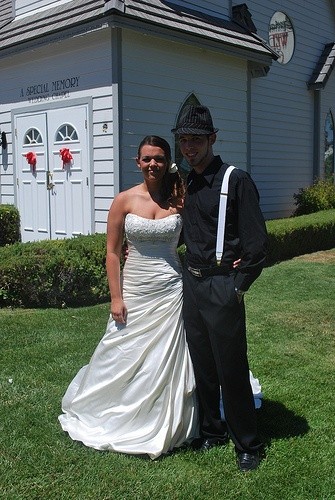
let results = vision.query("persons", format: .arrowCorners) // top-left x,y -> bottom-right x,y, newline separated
58,135 -> 261,461
122,106 -> 265,470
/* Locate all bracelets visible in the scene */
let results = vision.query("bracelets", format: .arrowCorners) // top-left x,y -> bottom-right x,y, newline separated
235,288 -> 245,295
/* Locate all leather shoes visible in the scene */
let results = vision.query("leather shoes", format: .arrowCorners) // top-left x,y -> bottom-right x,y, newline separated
198,437 -> 225,451
237,450 -> 258,468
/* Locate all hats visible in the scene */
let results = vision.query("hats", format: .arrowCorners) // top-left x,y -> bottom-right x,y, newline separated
172,104 -> 219,135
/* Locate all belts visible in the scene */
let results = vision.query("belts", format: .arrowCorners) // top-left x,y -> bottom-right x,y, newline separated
184,265 -> 228,275
